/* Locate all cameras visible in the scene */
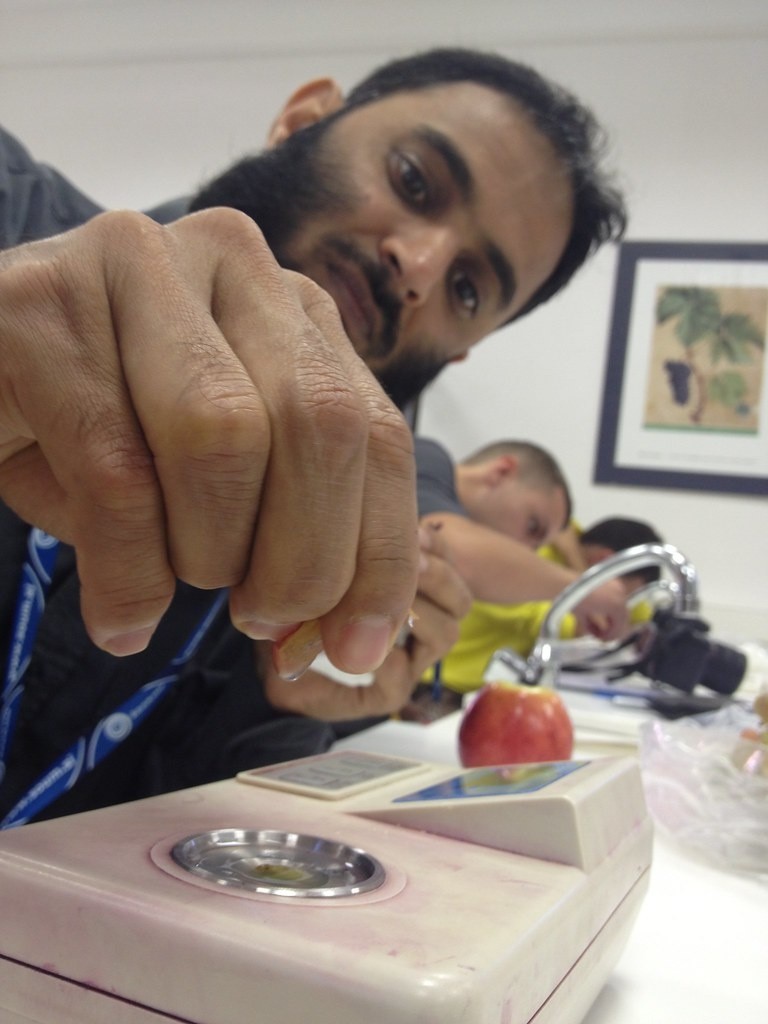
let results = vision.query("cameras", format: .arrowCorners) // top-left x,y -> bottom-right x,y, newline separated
638,608 -> 747,695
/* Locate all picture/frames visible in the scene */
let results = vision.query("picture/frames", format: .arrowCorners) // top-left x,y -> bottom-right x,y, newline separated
593,239 -> 768,496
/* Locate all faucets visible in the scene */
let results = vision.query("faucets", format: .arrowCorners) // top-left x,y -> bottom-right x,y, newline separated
542,542 -> 698,635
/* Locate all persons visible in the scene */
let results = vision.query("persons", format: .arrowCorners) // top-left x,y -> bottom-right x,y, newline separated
416,514 -> 663,705
337,434 -> 625,736
0,48 -> 625,829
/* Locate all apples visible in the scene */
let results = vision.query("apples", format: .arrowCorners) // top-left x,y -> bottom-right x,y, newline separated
457,680 -> 572,768
